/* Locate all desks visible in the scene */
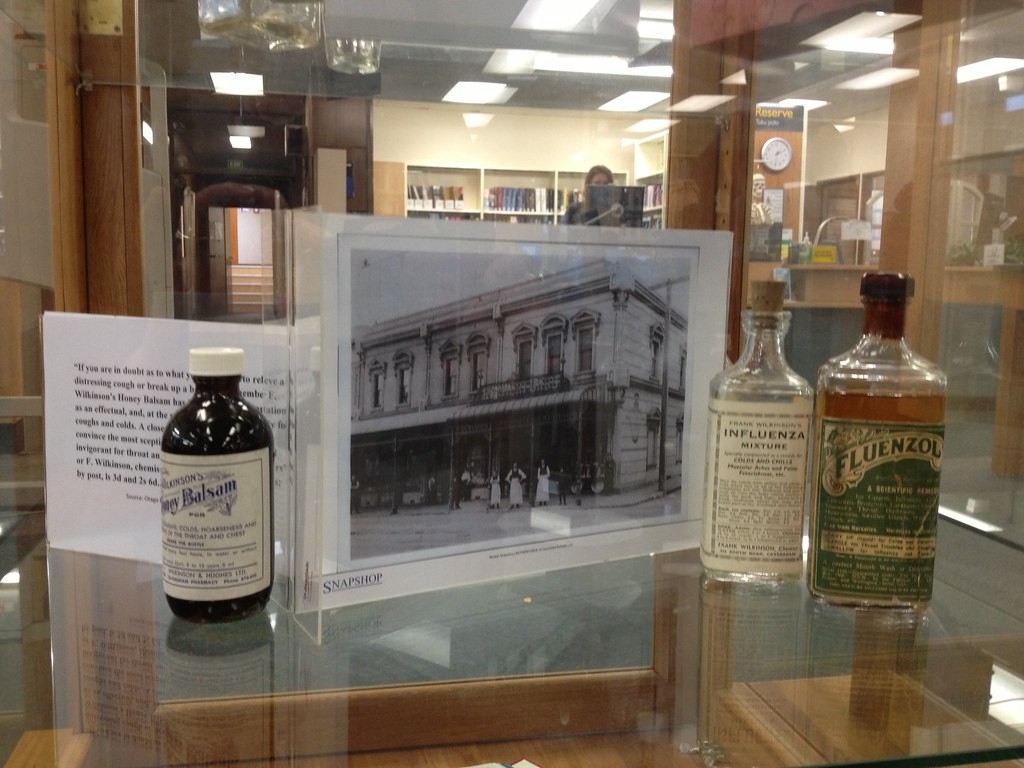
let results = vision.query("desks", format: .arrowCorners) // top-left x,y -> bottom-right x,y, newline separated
781,261 -> 1023,479
0,502 -> 1024,766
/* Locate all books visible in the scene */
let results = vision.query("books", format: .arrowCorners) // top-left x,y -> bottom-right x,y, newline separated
643,184 -> 664,208
406,185 -> 479,221
484,187 -> 583,224
641,216 -> 662,229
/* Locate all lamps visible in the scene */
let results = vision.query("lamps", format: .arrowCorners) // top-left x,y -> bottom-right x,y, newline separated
208,41 -> 267,140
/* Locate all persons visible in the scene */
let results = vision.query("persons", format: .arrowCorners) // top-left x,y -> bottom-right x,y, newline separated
490,470 -> 501,510
556,466 -> 569,504
350,476 -> 361,514
460,469 -> 471,501
561,165 -> 615,226
536,458 -> 550,505
428,476 -> 436,505
453,478 -> 461,509
505,461 -> 526,509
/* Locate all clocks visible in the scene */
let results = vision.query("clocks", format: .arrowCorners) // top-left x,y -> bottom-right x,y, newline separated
761,137 -> 794,173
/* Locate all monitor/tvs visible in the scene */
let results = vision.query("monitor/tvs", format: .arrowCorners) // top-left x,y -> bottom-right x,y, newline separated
584,184 -> 645,229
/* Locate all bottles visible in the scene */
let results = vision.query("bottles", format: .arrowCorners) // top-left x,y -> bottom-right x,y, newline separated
697,281 -> 805,585
807,271 -> 947,607
160,347 -> 274,621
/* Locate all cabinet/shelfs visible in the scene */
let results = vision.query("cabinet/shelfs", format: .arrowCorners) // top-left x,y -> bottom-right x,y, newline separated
371,158 -> 629,226
633,129 -> 670,230
